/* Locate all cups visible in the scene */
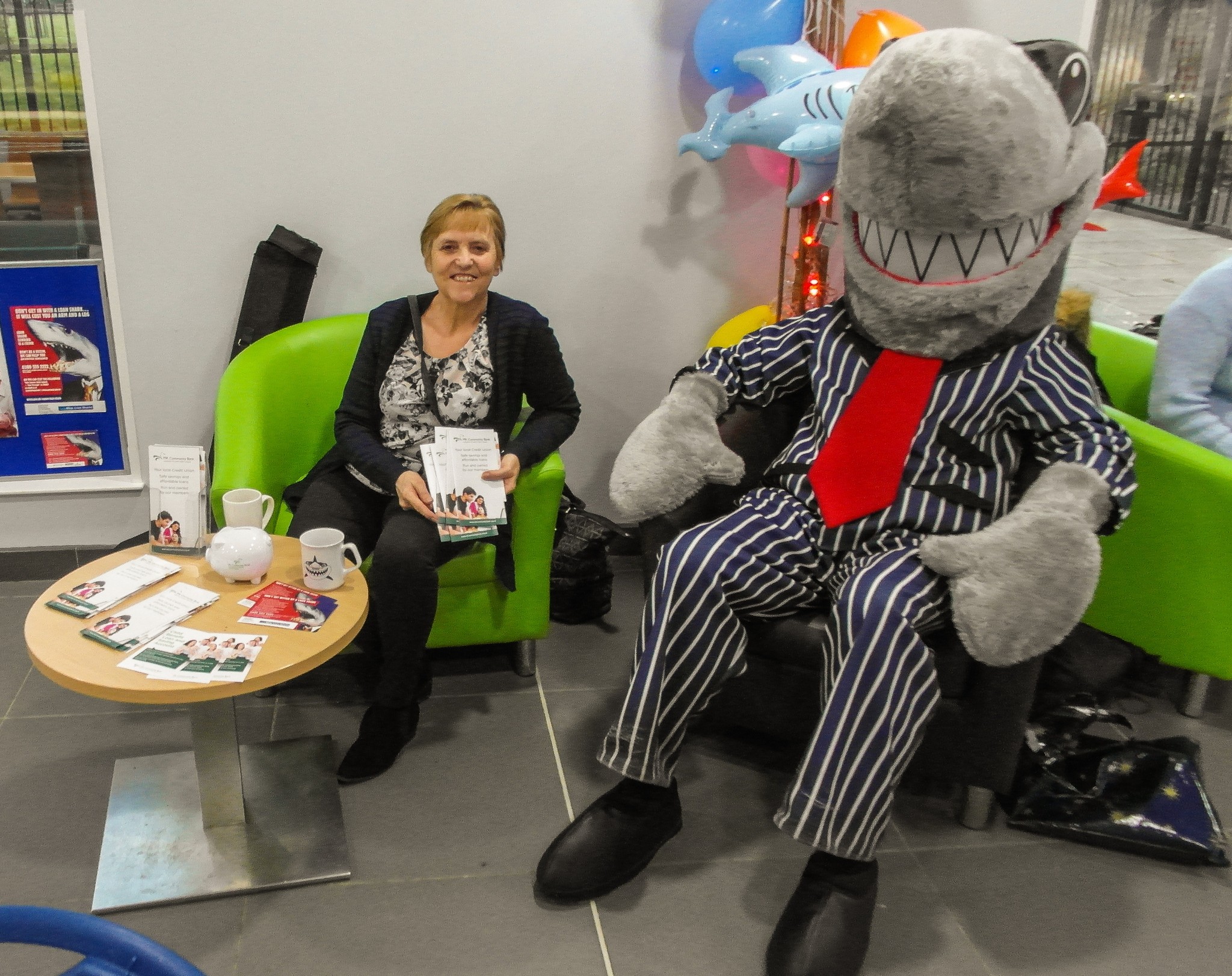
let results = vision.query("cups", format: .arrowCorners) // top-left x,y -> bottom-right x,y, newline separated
300,527 -> 362,591
222,489 -> 275,530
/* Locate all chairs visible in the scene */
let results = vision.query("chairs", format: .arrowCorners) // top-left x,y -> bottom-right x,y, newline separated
1042,307 -> 1231,721
204,310 -> 566,700
645,389 -> 1043,836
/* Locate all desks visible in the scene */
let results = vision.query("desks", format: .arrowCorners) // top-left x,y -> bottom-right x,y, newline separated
21,526 -> 374,912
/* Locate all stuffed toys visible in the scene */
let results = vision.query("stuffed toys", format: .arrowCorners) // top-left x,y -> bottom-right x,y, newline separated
536,26 -> 1139,976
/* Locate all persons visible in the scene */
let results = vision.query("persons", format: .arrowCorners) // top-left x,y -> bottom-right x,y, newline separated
282,193 -> 582,783
50,485 -> 488,663
1144,256 -> 1232,461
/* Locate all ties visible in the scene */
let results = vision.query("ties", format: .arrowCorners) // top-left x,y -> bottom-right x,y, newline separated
807,351 -> 946,527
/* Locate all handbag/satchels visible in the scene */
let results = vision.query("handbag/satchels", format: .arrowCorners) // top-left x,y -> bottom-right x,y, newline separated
548,484 -> 634,624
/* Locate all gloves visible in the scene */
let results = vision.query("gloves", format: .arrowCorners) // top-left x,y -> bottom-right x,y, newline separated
610,372 -> 747,524
918,459 -> 1114,667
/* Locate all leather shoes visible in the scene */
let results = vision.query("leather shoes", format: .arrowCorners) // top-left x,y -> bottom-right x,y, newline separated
766,853 -> 883,976
536,776 -> 685,899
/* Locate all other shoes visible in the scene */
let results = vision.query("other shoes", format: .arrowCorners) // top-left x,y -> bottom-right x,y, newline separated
339,702 -> 421,785
